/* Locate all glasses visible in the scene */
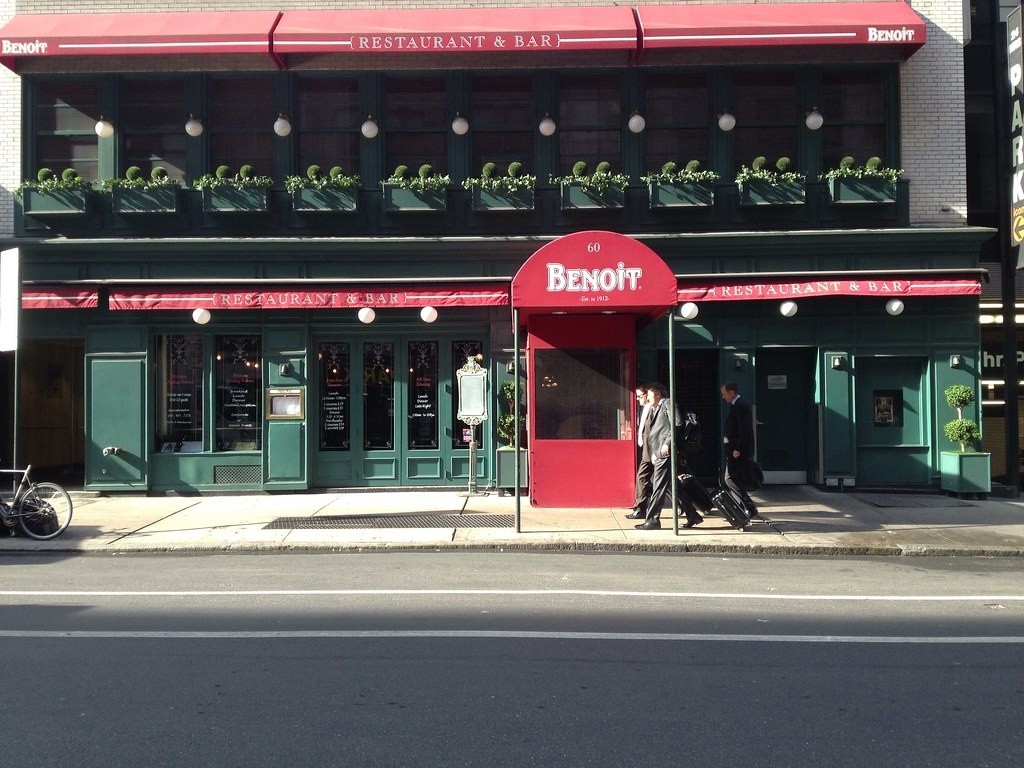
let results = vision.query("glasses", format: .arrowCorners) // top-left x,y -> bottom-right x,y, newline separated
637,393 -> 645,398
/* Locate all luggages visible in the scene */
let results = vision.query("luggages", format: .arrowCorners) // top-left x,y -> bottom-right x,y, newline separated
682,461 -> 752,532
690,476 -> 717,514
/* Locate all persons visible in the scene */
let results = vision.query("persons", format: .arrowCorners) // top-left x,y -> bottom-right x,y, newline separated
720,381 -> 757,521
634,384 -> 703,529
624,385 -> 690,519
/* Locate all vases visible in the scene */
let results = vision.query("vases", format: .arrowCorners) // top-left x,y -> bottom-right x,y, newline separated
23,177 -> 896,220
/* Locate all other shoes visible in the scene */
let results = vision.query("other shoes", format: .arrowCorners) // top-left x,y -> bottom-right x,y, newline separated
746,508 -> 758,518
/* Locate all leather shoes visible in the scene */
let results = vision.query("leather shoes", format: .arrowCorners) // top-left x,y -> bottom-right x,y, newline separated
634,521 -> 661,530
624,510 -> 647,519
678,508 -> 684,516
679,514 -> 703,528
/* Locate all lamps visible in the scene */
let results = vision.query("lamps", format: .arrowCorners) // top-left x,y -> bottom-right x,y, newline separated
628,110 -> 646,133
274,112 -> 292,136
358,307 -> 375,324
886,297 -> 904,315
805,105 -> 824,130
779,299 -> 798,317
191,309 -> 211,325
716,106 -> 736,131
361,114 -> 378,138
680,302 -> 698,319
95,115 -> 115,135
421,306 -> 438,323
452,112 -> 469,135
185,113 -> 203,136
539,113 -> 556,136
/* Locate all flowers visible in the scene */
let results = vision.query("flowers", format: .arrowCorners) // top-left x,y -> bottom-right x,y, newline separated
13,162 -> 904,199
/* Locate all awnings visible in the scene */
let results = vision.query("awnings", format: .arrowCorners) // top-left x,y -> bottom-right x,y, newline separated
0,2 -> 929,77
510,230 -> 679,537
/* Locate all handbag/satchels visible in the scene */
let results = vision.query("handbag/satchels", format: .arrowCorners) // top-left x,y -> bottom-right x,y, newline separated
726,452 -> 764,490
663,398 -> 702,455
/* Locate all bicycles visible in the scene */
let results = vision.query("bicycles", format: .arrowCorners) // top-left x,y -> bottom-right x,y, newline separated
0,465 -> 72,540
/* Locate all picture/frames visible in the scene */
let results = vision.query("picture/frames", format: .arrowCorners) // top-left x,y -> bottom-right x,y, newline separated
232,439 -> 260,451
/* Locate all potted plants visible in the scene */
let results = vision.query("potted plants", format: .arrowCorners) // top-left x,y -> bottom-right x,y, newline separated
495,380 -> 528,488
940,384 -> 991,492
